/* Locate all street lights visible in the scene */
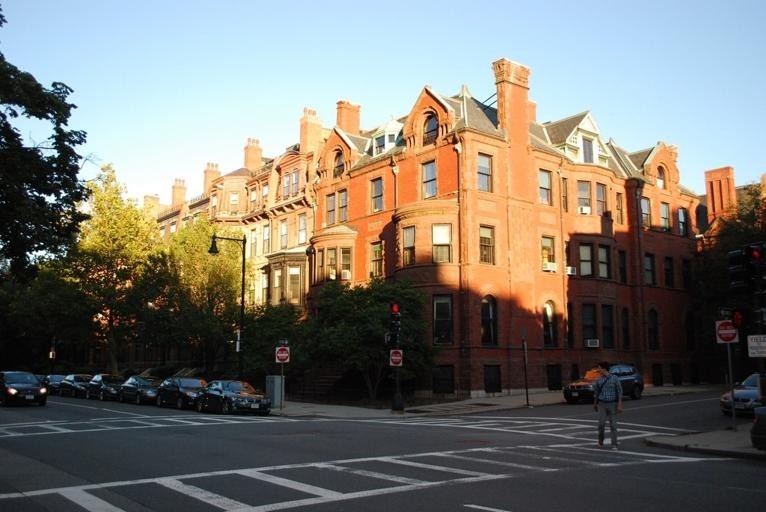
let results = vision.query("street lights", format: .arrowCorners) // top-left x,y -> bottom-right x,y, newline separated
206,232 -> 249,380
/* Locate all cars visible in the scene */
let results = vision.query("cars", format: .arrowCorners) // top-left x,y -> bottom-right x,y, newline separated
46,374 -> 66,395
156,376 -> 208,411
120,374 -> 164,405
0,371 -> 49,407
196,379 -> 271,417
88,373 -> 125,401
563,362 -> 645,404
61,373 -> 94,398
719,373 -> 761,416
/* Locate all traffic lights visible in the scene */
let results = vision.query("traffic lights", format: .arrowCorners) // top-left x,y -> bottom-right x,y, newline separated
727,239 -> 766,329
388,303 -> 403,321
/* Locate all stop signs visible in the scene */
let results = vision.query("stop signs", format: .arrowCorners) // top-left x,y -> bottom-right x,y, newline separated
714,318 -> 740,344
389,348 -> 403,367
275,346 -> 290,364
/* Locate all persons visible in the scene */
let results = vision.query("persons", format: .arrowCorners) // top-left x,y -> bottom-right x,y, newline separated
594,361 -> 623,450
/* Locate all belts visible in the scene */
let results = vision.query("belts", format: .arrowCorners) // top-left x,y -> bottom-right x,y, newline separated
599,399 -> 617,403
